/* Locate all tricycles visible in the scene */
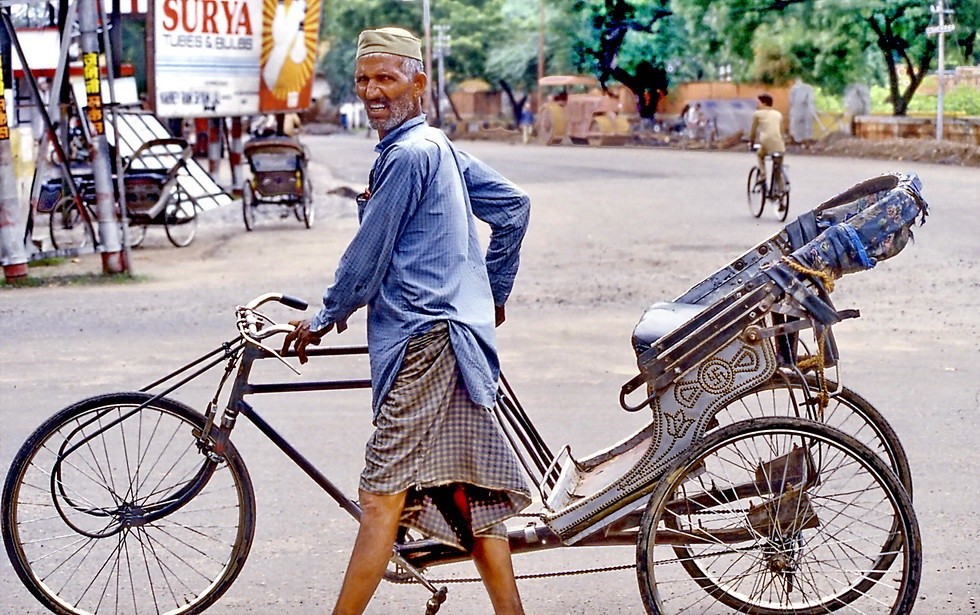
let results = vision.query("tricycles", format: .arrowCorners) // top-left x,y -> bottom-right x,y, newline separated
241,141 -> 316,231
48,137 -> 200,255
0,171 -> 930,615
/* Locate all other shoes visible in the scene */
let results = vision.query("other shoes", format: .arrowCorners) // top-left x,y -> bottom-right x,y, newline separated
756,179 -> 765,185
778,205 -> 786,211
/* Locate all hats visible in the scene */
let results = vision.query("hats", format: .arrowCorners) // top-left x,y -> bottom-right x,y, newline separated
356,27 -> 422,61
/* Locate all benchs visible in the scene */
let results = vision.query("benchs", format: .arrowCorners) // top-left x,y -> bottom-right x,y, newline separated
632,301 -> 804,416
117,167 -> 174,220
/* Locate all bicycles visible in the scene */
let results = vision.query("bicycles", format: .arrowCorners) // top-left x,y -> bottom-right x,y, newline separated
744,143 -> 791,222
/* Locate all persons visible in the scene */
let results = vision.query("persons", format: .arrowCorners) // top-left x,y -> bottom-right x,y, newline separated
252,108 -> 303,138
280,26 -> 532,615
518,103 -> 534,145
747,95 -> 787,211
681,103 -> 707,143
